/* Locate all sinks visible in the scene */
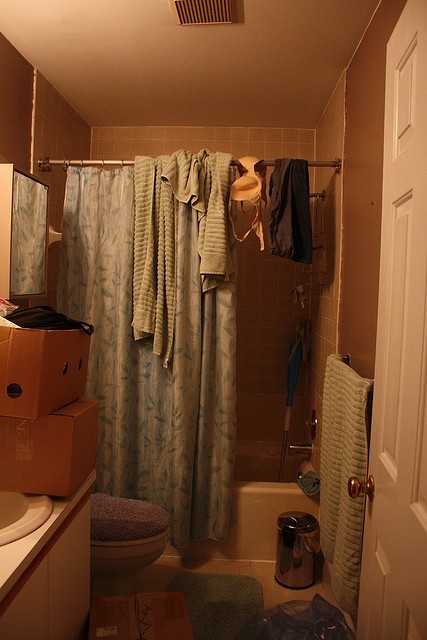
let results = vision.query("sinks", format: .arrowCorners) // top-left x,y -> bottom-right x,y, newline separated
0,491 -> 54,546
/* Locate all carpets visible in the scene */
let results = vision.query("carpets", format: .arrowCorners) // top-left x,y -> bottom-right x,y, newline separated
133,573 -> 264,640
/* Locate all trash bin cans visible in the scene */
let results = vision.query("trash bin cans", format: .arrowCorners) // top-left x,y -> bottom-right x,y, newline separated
276,510 -> 318,590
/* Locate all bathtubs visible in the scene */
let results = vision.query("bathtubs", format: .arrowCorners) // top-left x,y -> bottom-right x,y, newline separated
227,449 -> 320,560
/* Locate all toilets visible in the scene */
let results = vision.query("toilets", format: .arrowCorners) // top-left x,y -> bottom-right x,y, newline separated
90,494 -> 169,595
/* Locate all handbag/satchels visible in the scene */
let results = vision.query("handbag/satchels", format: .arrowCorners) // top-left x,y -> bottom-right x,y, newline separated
4,304 -> 93,335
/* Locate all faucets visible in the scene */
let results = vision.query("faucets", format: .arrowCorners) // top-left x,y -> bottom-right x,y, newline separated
288,443 -> 311,456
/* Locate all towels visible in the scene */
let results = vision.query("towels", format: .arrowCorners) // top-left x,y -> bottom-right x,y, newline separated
162,148 -> 233,292
319,354 -> 375,616
295,471 -> 321,497
131,155 -> 178,368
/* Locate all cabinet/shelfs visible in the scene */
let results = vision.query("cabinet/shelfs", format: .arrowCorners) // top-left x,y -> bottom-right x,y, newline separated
0,489 -> 91,640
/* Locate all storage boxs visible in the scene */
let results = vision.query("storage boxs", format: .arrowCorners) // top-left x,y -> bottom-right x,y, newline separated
90,591 -> 194,640
0,400 -> 99,497
1,326 -> 91,420
